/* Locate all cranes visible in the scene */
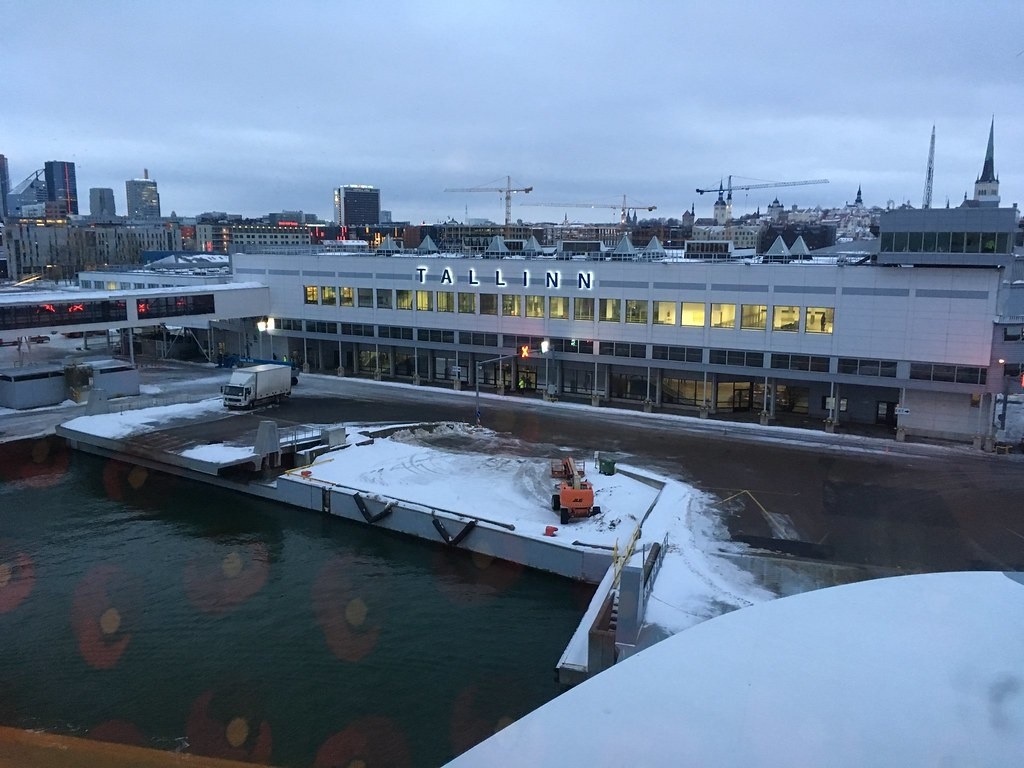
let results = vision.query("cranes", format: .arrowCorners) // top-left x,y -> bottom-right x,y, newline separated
443,175 -> 535,225
551,194 -> 656,224
695,174 -> 830,228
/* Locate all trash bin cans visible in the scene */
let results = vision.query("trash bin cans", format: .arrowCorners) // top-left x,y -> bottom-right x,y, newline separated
598,456 -> 616,476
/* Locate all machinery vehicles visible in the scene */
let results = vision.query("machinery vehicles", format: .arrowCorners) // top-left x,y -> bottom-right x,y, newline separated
550,455 -> 600,524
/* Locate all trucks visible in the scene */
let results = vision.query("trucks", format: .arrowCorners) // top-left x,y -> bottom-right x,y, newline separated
220,363 -> 292,412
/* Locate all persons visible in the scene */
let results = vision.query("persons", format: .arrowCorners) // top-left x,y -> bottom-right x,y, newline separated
528,377 -> 533,389
521,374 -> 526,387
820,313 -> 827,332
